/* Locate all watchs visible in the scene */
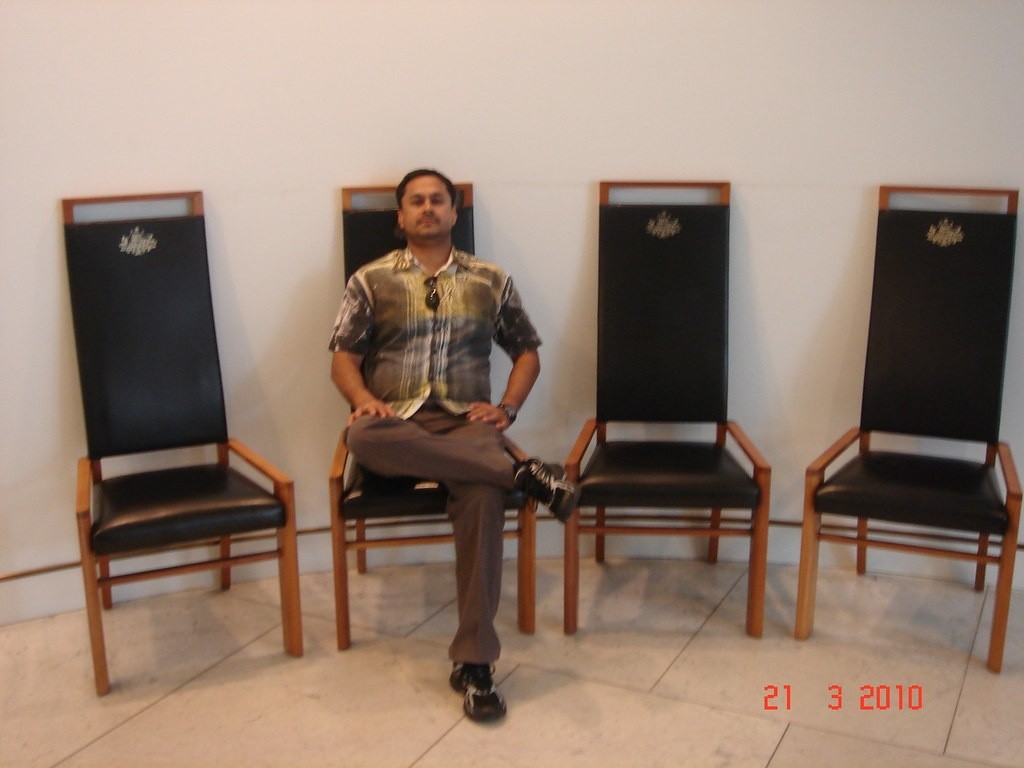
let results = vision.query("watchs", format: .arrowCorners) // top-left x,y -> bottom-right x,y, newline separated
499,403 -> 517,421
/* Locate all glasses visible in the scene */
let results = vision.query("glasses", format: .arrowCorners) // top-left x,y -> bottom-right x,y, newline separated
424,276 -> 440,311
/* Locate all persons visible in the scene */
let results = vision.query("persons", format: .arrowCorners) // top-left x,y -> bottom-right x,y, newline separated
328,168 -> 578,720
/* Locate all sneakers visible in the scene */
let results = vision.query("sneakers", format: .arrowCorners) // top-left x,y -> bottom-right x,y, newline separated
524,452 -> 575,522
449,661 -> 507,723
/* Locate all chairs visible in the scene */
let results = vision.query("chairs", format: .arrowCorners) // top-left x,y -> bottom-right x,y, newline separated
558,180 -> 772,641
57,190 -> 304,697
327,181 -> 542,648
792,186 -> 1023,673
